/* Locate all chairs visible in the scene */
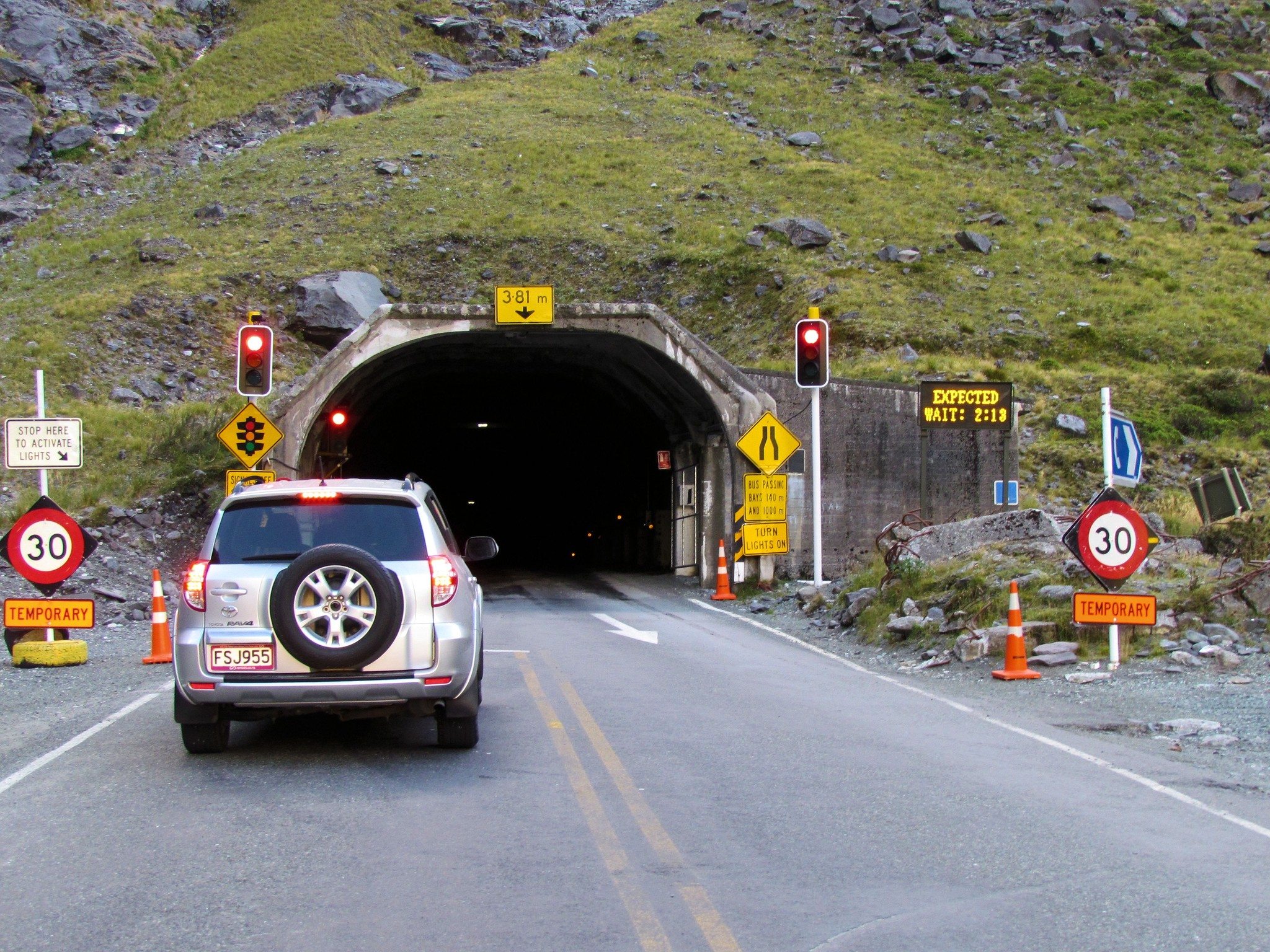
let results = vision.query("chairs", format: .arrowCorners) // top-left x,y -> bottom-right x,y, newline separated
261,513 -> 313,555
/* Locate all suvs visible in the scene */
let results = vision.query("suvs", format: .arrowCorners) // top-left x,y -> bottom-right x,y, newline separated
171,473 -> 499,754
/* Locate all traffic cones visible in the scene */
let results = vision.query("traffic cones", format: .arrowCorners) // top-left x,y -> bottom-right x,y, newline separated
709,539 -> 737,600
141,569 -> 175,665
992,582 -> 1041,679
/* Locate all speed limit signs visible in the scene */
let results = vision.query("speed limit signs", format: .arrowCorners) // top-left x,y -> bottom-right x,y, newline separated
11,508 -> 84,583
1079,505 -> 1148,579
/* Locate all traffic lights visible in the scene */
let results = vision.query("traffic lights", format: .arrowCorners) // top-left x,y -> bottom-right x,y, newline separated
238,326 -> 271,396
328,407 -> 347,457
795,319 -> 829,388
